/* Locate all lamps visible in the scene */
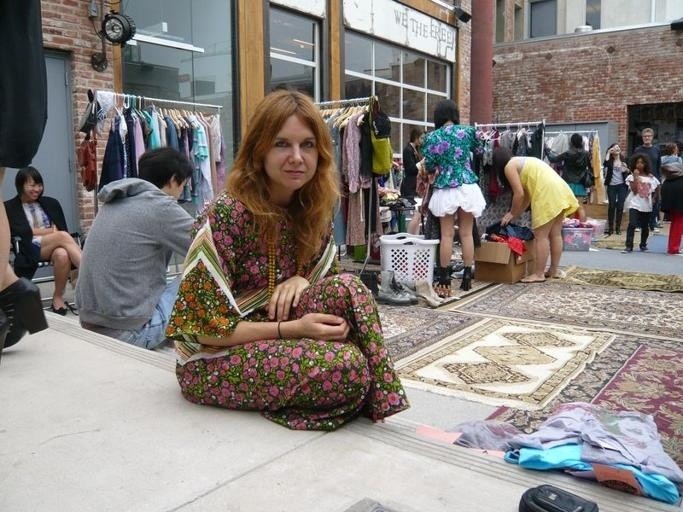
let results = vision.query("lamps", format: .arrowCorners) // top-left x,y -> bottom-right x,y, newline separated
85,0 -> 137,76
445,4 -> 472,23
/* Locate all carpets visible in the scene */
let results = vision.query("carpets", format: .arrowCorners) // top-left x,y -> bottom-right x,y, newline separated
584,212 -> 675,253
364,261 -> 683,410
453,334 -> 683,470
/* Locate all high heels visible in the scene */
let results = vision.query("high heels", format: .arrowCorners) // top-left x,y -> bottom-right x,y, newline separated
435,267 -> 452,293
462,267 -> 472,291
0,276 -> 49,365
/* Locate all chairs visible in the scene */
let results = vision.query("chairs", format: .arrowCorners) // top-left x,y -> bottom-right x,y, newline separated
5,229 -> 83,311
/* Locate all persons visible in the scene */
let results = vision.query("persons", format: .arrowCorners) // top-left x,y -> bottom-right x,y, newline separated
4,166 -> 84,317
0,0 -> 48,354
73,148 -> 202,350
164,89 -> 410,432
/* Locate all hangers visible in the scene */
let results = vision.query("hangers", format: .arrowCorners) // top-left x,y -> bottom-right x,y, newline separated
312,95 -> 372,133
554,129 -> 600,144
80,90 -> 222,140
470,120 -> 541,143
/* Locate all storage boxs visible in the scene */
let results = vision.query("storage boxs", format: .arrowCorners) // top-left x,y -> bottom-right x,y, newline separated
579,216 -> 607,243
559,227 -> 592,252
474,237 -> 537,285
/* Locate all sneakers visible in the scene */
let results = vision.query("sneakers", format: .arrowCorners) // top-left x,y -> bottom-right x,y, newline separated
640,246 -> 652,253
621,247 -> 633,254
52,304 -> 67,317
667,249 -> 683,256
634,227 -> 640,232
649,221 -> 664,234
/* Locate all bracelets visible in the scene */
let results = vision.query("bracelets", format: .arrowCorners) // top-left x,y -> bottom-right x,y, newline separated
278,320 -> 283,339
